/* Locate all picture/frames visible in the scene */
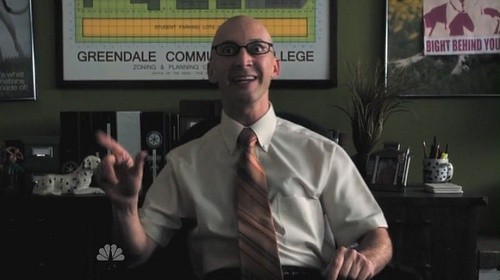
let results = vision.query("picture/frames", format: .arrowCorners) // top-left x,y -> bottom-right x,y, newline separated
372,148 -> 400,190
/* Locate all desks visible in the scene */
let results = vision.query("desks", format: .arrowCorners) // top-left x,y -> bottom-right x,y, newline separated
0,183 -> 489,279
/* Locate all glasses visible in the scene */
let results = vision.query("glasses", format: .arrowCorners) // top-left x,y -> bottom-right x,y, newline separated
210,40 -> 276,57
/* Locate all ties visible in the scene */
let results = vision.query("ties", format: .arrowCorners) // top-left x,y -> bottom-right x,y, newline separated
236,127 -> 284,280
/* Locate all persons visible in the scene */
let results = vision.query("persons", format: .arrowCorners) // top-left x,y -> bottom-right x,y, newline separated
92,14 -> 393,280
377,156 -> 398,184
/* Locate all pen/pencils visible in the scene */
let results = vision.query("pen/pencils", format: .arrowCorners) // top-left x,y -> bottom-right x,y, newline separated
422,134 -> 449,158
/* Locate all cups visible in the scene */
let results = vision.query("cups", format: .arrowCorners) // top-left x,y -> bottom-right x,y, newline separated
423,159 -> 453,184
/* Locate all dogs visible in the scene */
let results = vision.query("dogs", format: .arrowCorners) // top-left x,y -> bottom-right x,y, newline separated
31,155 -> 105,197
